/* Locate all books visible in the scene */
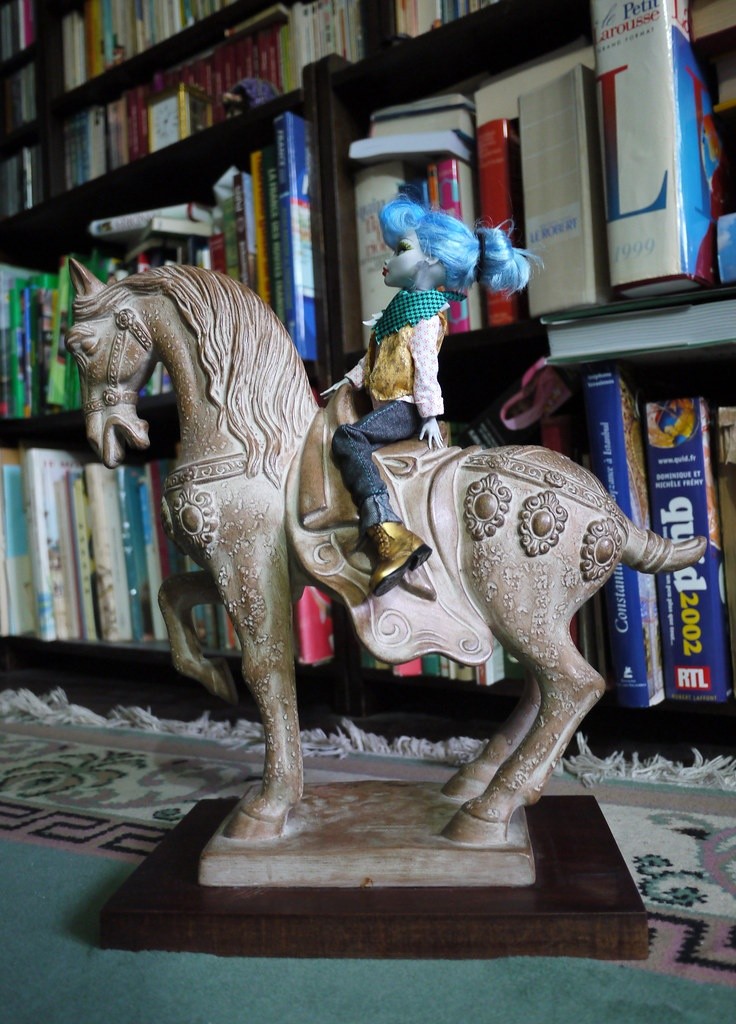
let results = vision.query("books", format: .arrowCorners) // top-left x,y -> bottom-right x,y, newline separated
0,0 -> 736,710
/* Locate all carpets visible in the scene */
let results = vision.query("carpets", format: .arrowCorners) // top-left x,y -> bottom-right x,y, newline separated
2,688 -> 734,1023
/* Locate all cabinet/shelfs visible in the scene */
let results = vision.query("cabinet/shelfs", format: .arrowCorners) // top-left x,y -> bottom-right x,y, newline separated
2,0 -> 736,764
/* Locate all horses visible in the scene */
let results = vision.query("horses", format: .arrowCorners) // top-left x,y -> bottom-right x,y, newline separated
63,256 -> 711,845
